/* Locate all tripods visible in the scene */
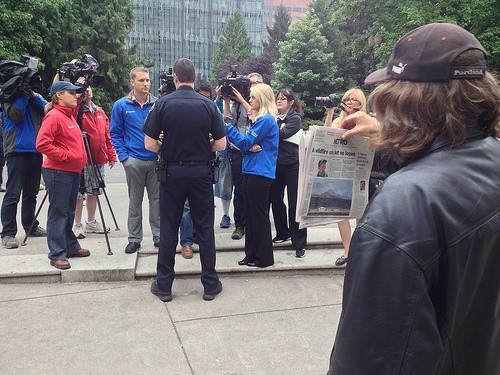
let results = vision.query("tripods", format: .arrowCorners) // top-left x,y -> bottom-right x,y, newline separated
22,100 -> 121,256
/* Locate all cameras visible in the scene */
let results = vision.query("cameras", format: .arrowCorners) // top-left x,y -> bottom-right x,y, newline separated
314,93 -> 340,108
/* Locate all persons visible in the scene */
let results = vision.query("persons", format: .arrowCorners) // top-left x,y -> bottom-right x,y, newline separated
0,86 -> 49,249
110,67 -> 160,253
323,89 -> 367,266
327,20 -> 500,375
222,83 -> 279,267
72,86 -> 117,239
0,98 -> 7,192
180,80 -> 211,260
224,72 -> 267,240
272,89 -> 307,258
213,85 -> 236,228
141,57 -> 226,302
317,159 -> 329,178
35,81 -> 90,269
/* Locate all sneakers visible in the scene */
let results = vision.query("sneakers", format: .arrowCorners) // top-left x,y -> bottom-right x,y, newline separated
295,249 -> 307,258
1,235 -> 18,249
151,281 -> 172,302
203,281 -> 222,300
49,259 -> 71,269
231,226 -> 245,239
220,214 -> 231,229
335,255 -> 348,266
84,220 -> 111,234
73,223 -> 85,239
273,236 -> 291,243
27,226 -> 48,237
71,248 -> 90,257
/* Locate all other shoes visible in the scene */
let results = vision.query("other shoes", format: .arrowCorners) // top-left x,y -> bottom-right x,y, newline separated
239,257 -> 256,267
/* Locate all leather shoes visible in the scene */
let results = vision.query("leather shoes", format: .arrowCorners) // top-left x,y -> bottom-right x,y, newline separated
182,245 -> 193,259
155,242 -> 160,246
125,242 -> 141,253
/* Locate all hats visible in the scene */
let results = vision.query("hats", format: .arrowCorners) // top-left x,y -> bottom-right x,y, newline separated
51,81 -> 84,96
365,20 -> 489,85
